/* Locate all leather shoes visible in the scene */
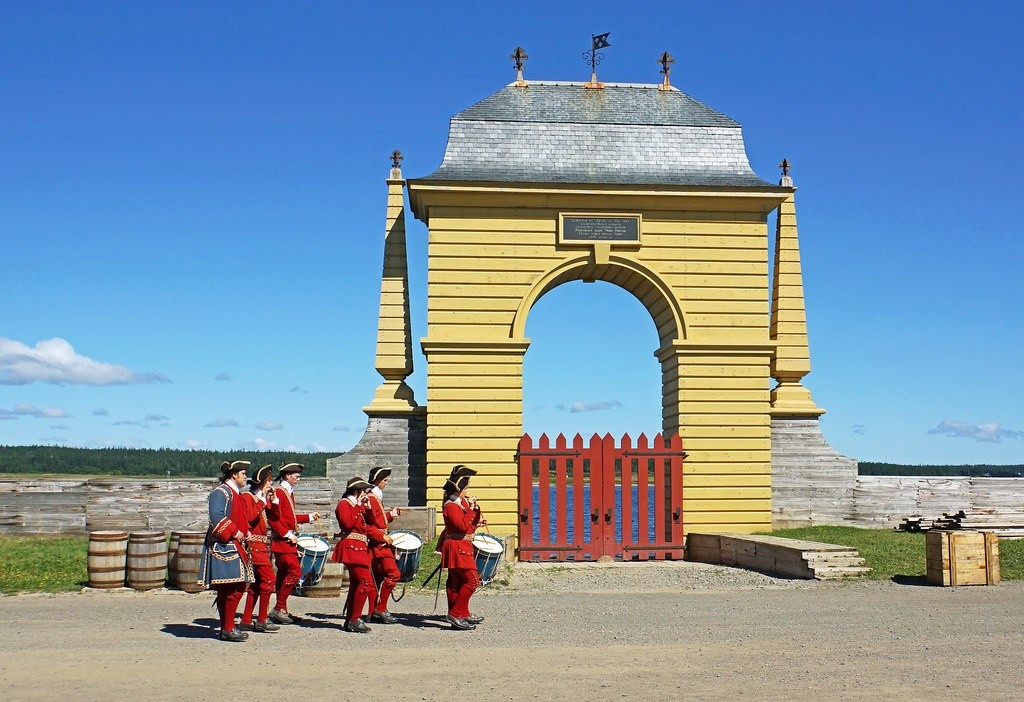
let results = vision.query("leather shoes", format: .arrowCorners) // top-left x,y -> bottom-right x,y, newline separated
466,616 -> 484,623
344,617 -> 371,632
239,621 -> 256,630
220,628 -> 248,639
270,608 -> 294,623
445,613 -> 476,629
255,618 -> 280,631
374,609 -> 399,623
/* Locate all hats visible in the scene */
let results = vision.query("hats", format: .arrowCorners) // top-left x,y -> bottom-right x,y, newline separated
450,465 -> 477,476
346,477 -> 375,489
369,467 -> 391,481
220,460 -> 250,474
442,476 -> 469,492
278,463 -> 304,472
246,464 -> 271,486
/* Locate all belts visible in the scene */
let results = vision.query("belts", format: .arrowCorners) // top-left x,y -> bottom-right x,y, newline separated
341,532 -> 368,543
377,528 -> 388,535
271,530 -> 298,538
444,532 -> 474,542
244,534 -> 268,543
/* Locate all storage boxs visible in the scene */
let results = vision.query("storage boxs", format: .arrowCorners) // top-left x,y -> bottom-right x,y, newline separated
926,530 -> 1001,587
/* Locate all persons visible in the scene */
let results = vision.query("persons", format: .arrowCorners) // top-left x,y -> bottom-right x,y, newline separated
362,467 -> 402,624
435,464 -> 487,629
268,463 -> 320,625
332,478 -> 376,633
197,461 -> 253,641
239,464 -> 280,632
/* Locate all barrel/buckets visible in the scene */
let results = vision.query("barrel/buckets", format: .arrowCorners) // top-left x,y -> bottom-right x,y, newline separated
175,532 -> 207,593
168,531 -> 201,589
87,530 -> 129,588
267,527 -> 350,598
126,531 -> 168,590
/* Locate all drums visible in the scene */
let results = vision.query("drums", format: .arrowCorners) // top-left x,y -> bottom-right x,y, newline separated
294,532 -> 332,586
472,532 -> 504,587
388,528 -> 423,583
